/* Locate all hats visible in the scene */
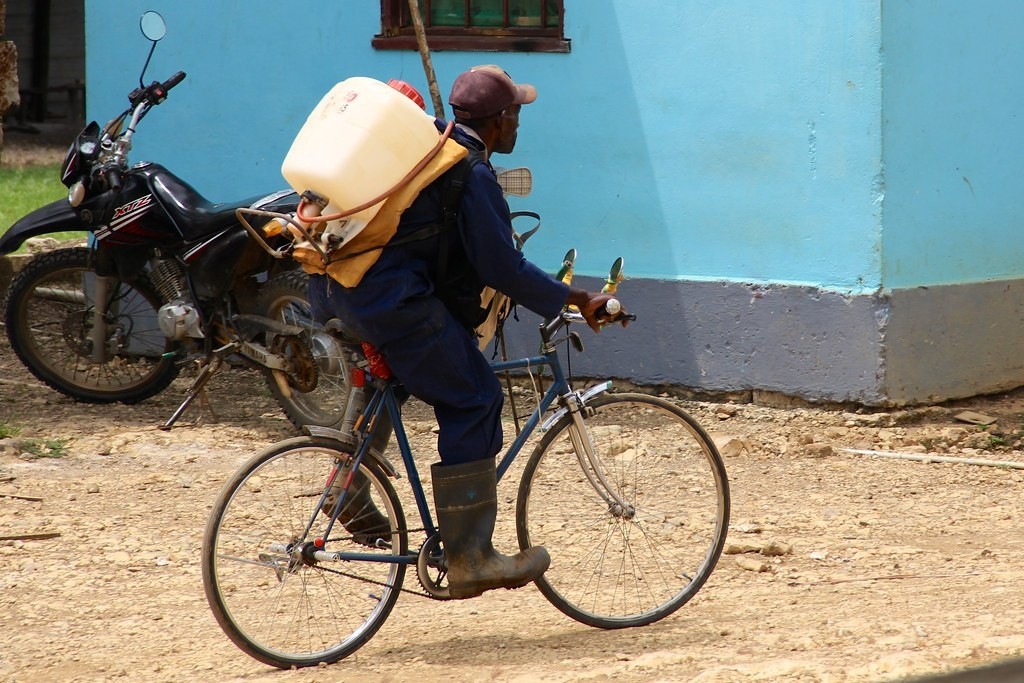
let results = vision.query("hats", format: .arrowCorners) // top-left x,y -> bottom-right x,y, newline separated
449,66 -> 538,121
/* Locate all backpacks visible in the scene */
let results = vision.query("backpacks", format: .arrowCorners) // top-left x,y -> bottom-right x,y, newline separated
455,210 -> 543,353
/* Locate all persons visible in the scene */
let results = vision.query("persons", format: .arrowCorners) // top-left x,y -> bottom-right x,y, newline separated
303,63 -> 633,602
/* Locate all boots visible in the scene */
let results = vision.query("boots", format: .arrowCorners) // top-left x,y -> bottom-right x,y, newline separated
431,462 -> 551,600
318,369 -> 394,546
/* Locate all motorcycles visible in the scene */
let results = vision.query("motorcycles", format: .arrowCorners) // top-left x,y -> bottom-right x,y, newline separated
0,9 -> 357,437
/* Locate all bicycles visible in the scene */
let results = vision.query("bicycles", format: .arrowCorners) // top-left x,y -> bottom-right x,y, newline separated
201,244 -> 732,670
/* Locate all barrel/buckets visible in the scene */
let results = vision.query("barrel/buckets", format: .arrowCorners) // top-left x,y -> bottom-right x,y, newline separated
282,77 -> 442,220
